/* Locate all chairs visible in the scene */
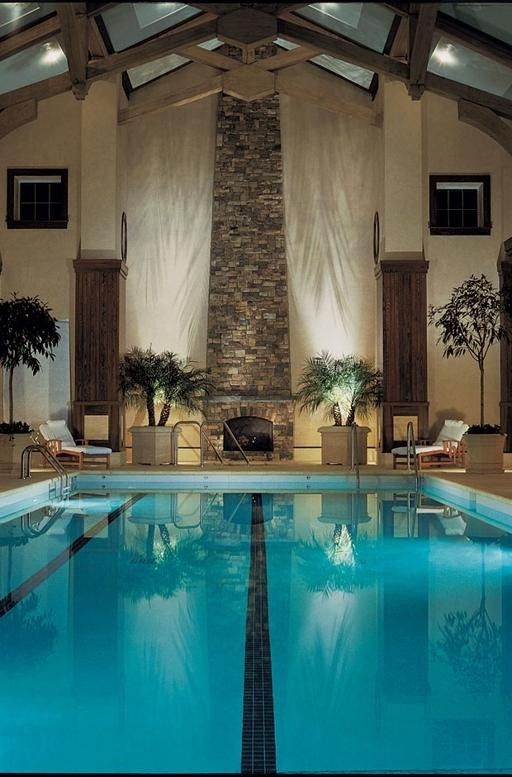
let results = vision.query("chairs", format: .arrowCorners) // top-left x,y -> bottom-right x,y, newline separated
390,493 -> 467,538
36,419 -> 112,471
391,419 -> 471,471
36,491 -> 127,535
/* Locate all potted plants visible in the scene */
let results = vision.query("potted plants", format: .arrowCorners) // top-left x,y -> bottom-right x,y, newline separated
0,515 -> 58,671
293,350 -> 386,468
429,507 -> 509,699
292,492 -> 383,601
111,491 -> 211,609
0,290 -> 63,476
426,272 -> 512,474
117,343 -> 216,466
